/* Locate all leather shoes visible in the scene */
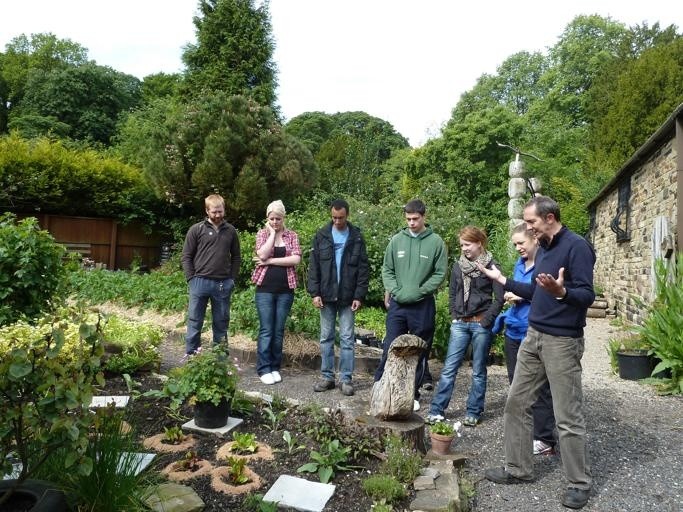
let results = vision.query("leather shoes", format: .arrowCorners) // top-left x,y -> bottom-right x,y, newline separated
485,466 -> 537,485
561,482 -> 591,509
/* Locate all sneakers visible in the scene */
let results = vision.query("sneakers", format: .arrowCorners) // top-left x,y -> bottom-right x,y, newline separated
313,378 -> 336,392
532,437 -> 556,455
422,383 -> 433,391
423,413 -> 446,424
463,414 -> 479,427
258,370 -> 283,385
412,398 -> 421,411
337,379 -> 354,397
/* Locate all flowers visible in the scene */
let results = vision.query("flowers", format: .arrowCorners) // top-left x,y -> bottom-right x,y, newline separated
164,336 -> 243,410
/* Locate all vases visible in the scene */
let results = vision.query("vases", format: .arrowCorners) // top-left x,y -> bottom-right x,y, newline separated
192,401 -> 231,429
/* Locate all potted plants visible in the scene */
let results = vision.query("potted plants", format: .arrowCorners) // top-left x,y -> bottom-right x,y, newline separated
616,329 -> 672,381
0,307 -> 117,512
486,342 -> 503,366
428,421 -> 456,456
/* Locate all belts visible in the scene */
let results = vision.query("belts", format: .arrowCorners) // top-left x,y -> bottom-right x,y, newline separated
462,314 -> 480,323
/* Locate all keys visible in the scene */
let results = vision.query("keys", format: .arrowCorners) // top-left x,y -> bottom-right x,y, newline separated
218,280 -> 224,292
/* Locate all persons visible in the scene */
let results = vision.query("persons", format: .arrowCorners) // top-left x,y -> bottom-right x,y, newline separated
490,223 -> 556,456
307,199 -> 369,396
425,225 -> 505,427
472,196 -> 597,510
249,200 -> 302,386
383,290 -> 434,389
372,198 -> 447,411
182,193 -> 241,361
